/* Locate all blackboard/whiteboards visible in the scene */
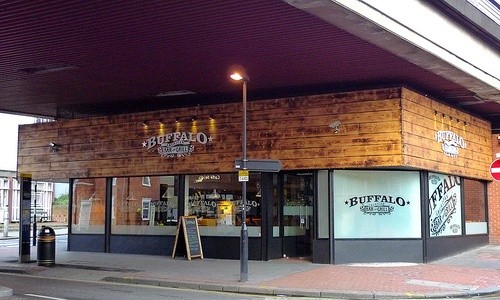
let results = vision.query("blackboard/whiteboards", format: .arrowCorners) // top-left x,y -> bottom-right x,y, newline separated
171,215 -> 204,258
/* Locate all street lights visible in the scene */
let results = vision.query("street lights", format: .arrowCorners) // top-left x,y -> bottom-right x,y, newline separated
231,67 -> 250,282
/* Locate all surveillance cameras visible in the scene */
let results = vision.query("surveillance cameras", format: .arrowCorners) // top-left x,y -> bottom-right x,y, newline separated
329,119 -> 341,134
49,142 -> 57,152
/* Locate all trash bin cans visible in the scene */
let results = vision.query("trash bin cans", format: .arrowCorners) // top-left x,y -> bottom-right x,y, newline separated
36,226 -> 56,266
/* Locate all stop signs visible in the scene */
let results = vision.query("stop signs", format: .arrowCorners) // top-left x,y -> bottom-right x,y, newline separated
490,159 -> 500,182
